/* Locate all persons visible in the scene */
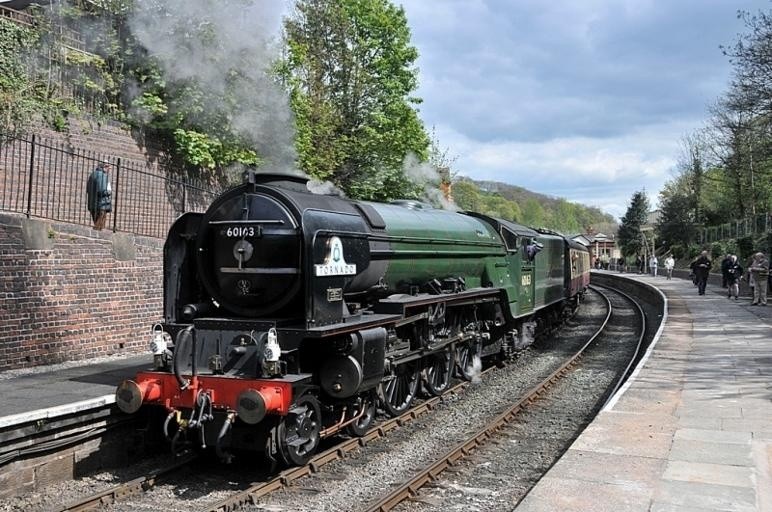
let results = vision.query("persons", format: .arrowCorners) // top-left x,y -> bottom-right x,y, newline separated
87,159 -> 112,230
527,243 -> 544,260
635,253 -> 658,276
721,251 -> 772,306
572,251 -> 578,263
591,254 -> 600,269
619,256 -> 624,273
664,253 -> 674,279
690,250 -> 711,295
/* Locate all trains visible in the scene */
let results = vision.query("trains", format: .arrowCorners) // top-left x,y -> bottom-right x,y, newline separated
115,169 -> 591,471
